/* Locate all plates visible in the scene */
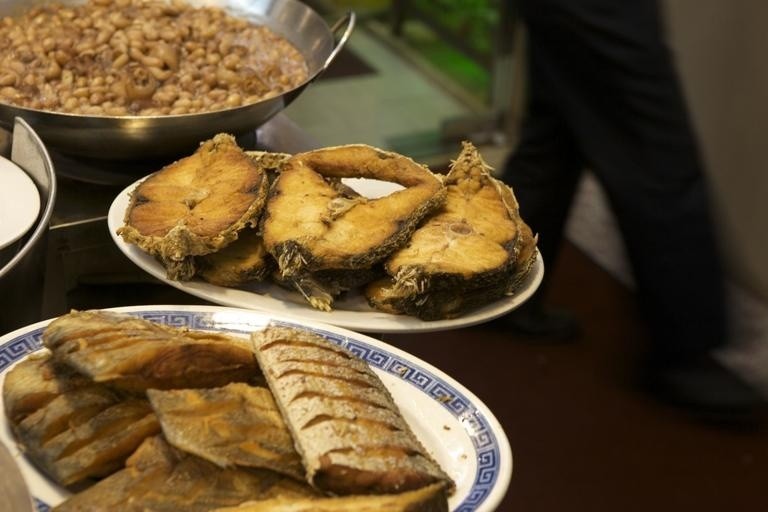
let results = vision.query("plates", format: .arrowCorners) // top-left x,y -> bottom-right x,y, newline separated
0,304 -> 515,512
0,154 -> 42,251
106,152 -> 545,332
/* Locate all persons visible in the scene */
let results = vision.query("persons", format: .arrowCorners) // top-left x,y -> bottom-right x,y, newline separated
479,2 -> 767,434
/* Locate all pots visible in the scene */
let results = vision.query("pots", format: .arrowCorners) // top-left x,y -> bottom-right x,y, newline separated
0,0 -> 357,186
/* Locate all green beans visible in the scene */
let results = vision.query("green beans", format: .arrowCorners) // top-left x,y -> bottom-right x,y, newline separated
0,0 -> 306,115
